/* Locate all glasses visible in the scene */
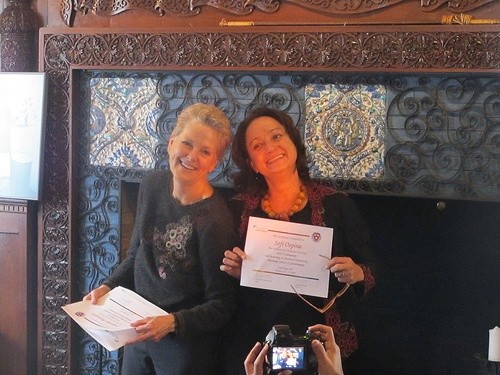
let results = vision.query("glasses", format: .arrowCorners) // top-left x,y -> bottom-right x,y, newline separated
291,255 -> 350,313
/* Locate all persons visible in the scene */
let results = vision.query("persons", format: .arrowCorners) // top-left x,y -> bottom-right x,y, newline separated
244,323 -> 345,375
213,107 -> 384,375
83,104 -> 241,375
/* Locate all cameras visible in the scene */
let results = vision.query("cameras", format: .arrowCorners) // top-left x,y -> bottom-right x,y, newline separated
261,325 -> 326,375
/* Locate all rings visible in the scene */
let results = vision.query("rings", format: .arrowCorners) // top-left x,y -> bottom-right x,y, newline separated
340,272 -> 345,276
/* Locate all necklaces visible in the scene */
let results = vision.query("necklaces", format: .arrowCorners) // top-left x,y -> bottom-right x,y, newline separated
263,185 -> 306,220
